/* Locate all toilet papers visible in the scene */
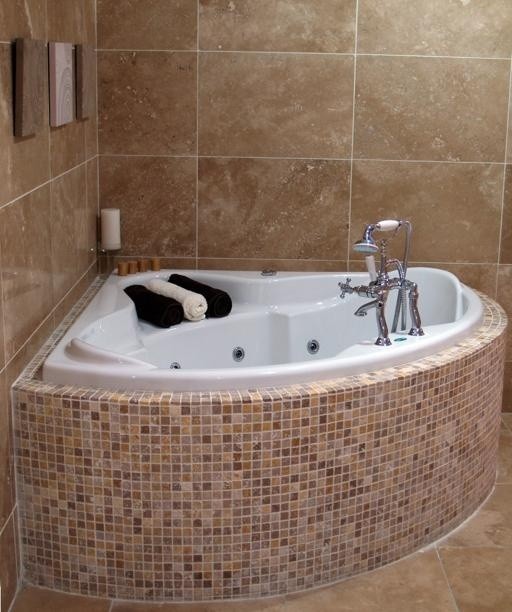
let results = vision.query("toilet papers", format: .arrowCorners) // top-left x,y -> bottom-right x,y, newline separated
100,208 -> 121,251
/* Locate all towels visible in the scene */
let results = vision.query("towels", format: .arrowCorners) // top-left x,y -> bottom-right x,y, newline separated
122,273 -> 232,328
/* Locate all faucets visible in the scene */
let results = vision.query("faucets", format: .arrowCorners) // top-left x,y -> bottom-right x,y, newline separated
377,256 -> 408,330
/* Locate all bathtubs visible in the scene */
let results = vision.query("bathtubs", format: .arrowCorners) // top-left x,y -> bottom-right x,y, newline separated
38,261 -> 485,389
